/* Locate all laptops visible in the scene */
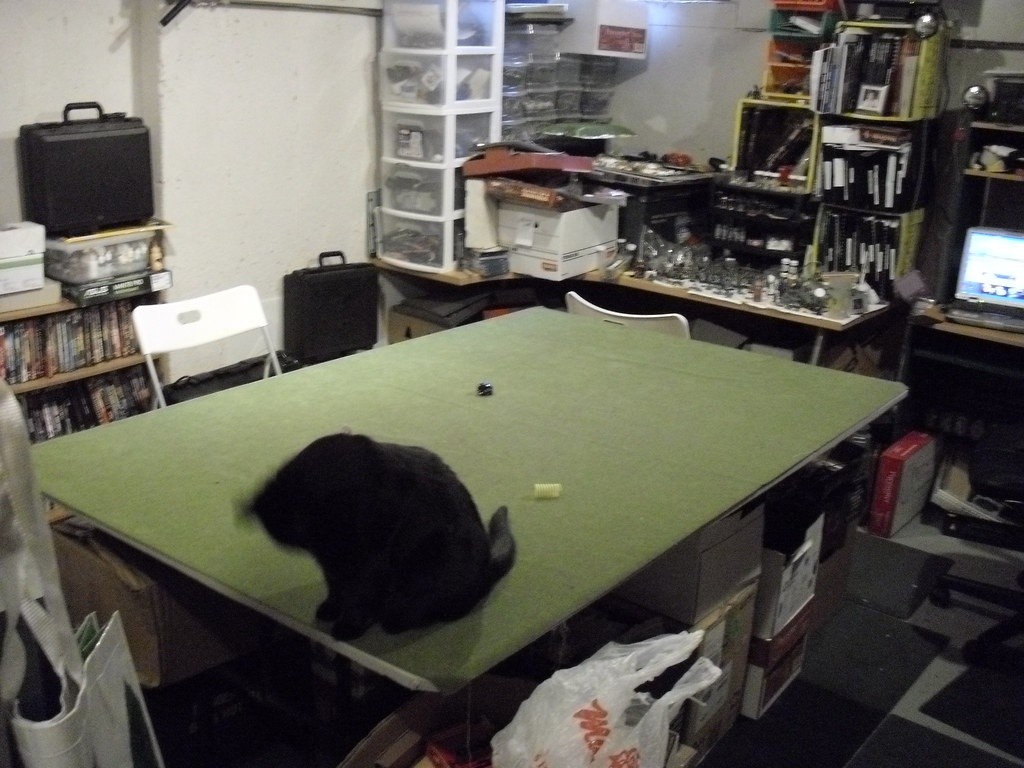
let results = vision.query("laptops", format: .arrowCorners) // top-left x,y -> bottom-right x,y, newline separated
946,227 -> 1024,333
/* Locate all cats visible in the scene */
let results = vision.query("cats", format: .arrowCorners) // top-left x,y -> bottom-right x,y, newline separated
234,427 -> 516,644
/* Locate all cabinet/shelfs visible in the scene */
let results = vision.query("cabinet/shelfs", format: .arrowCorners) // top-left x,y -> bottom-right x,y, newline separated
962,66 -> 1024,226
372,0 -> 505,278
731,0 -> 954,281
0,289 -> 168,444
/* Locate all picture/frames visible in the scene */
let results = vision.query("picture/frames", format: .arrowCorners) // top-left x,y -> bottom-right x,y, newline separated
856,82 -> 889,115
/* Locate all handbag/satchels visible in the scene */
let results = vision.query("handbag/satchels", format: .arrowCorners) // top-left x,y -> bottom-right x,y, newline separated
0,537 -> 166,768
282,251 -> 380,360
490,629 -> 722,768
19,101 -> 154,240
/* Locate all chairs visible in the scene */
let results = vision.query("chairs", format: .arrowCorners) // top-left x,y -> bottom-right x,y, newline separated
132,285 -> 281,409
565,291 -> 690,337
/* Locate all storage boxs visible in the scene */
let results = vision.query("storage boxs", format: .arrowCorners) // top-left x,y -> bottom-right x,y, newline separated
503,0 -> 649,141
336,431 -> 879,768
499,203 -> 618,256
388,289 -> 553,342
512,242 -> 618,282
578,172 -> 716,244
867,428 -> 942,538
48,512 -> 302,768
0,221 -> 172,308
942,508 -> 1024,551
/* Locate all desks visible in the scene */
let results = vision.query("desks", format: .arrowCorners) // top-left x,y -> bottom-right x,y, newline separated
371,258 -> 1024,382
30,306 -> 908,692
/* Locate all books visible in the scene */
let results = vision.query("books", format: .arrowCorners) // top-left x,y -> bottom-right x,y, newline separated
0,294 -> 146,385
824,210 -> 900,301
809,33 -> 921,118
15,363 -> 158,444
736,106 -> 814,176
822,144 -> 912,214
